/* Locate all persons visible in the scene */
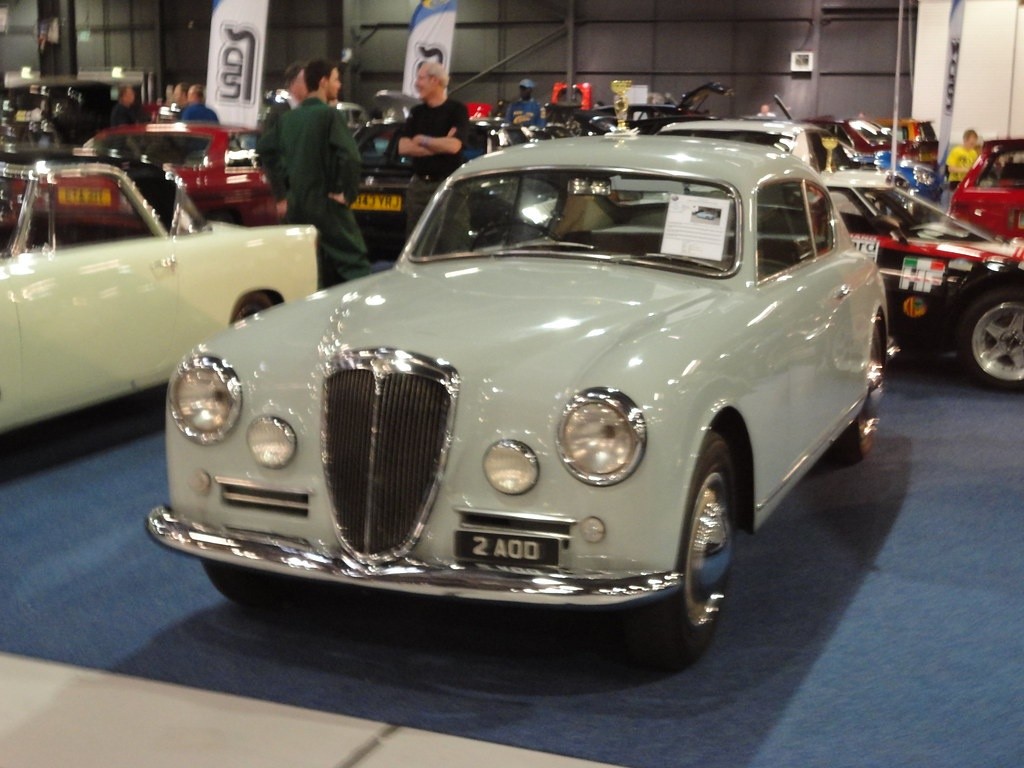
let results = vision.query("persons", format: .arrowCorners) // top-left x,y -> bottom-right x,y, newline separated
506,79 -> 546,129
104,82 -> 220,126
757,104 -> 776,120
394,59 -> 474,254
258,58 -> 377,290
948,127 -> 982,191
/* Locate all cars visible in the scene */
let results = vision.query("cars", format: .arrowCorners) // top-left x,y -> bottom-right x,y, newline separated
1,141 -> 322,439
809,115 -> 921,170
812,170 -> 1023,392
840,144 -> 945,200
143,135 -> 900,670
950,138 -> 1024,244
1,123 -> 290,227
571,82 -> 730,137
333,124 -> 561,260
605,118 -> 863,206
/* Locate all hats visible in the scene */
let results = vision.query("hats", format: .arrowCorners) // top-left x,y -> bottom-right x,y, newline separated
518,78 -> 534,89
